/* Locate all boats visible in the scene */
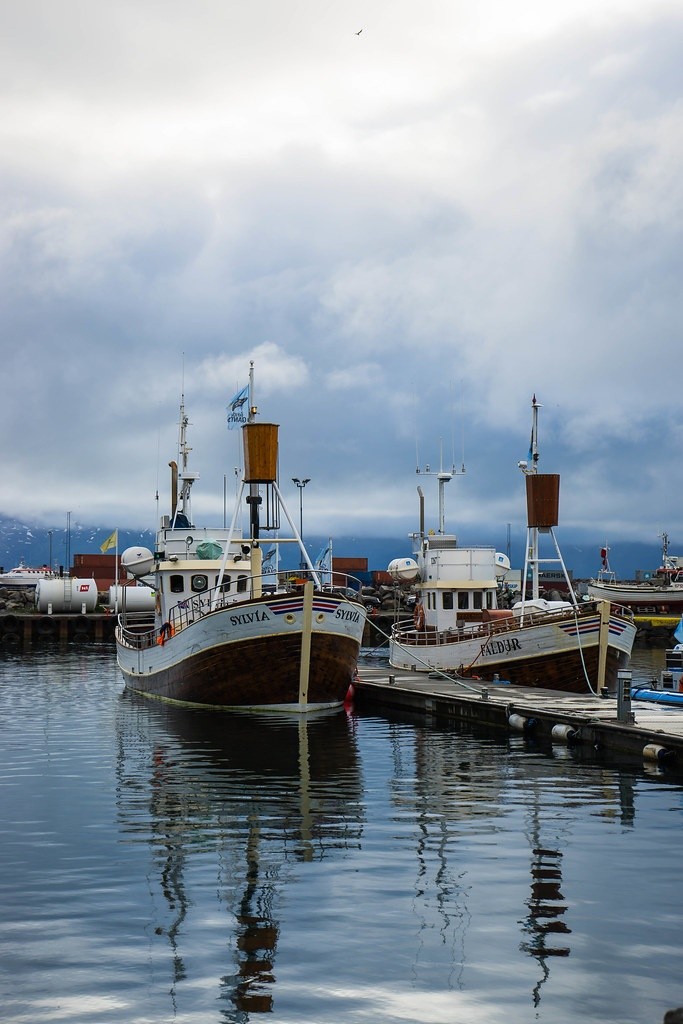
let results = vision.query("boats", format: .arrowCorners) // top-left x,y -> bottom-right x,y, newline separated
632,643 -> 683,705
0,557 -> 61,589
116,362 -> 368,717
387,395 -> 635,702
587,532 -> 683,641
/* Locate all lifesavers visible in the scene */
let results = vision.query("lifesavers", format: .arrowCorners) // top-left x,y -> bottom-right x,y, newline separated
414,605 -> 424,630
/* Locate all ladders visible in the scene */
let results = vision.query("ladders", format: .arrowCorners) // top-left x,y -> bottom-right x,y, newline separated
62,575 -> 73,613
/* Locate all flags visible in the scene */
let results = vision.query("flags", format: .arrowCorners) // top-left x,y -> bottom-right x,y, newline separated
262,534 -> 282,583
226,384 -> 249,430
100,531 -> 116,554
314,543 -> 331,583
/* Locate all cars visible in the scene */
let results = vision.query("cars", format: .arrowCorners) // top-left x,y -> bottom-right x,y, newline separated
406,597 -> 419,609
319,586 -> 380,614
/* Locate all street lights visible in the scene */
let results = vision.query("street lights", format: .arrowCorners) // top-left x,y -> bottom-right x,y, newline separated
293,478 -> 311,579
